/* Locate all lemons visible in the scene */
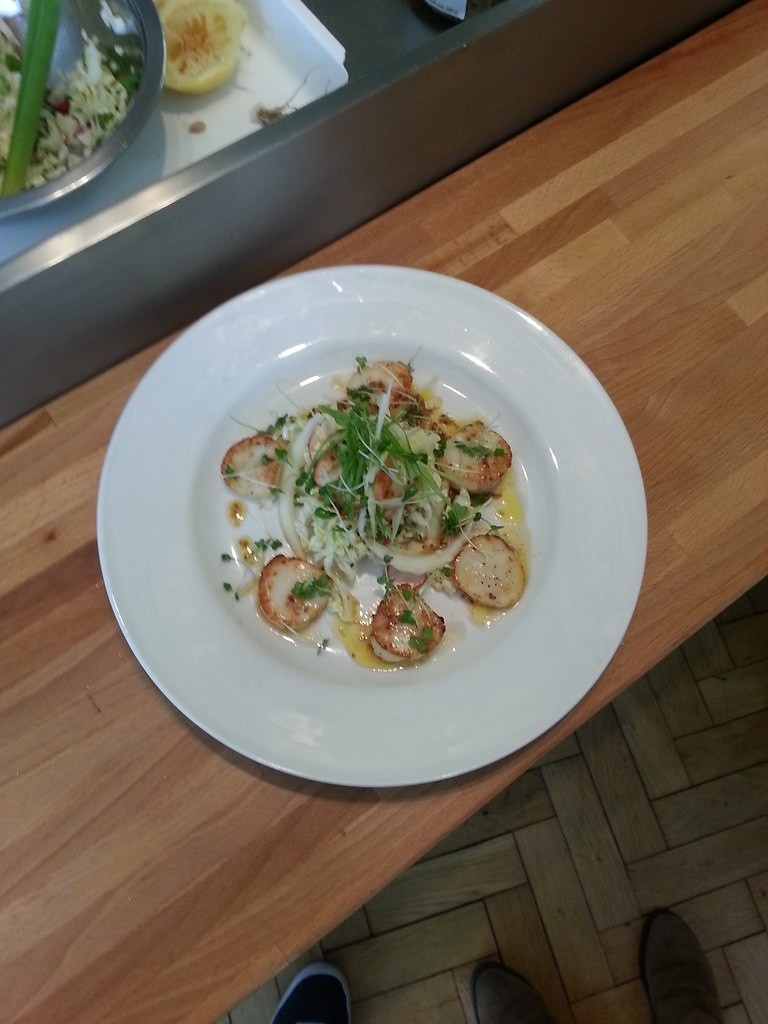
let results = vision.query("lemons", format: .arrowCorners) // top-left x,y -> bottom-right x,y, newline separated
155,0 -> 242,95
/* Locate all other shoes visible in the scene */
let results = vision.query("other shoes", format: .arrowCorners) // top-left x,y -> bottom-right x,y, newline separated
639,908 -> 726,1024
470,960 -> 555,1024
268,962 -> 351,1024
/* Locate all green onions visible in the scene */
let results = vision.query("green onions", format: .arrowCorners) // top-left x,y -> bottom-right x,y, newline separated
0,0 -> 69,199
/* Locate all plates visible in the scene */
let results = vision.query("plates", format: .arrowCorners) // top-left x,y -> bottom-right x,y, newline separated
96,265 -> 647,788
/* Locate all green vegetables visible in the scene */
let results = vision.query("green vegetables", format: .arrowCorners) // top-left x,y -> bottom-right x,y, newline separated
219,355 -> 508,652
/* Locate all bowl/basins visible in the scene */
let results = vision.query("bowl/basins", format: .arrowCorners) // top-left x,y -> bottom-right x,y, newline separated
0,0 -> 167,219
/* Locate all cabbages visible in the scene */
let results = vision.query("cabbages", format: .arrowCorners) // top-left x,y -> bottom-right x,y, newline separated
1,30 -> 131,189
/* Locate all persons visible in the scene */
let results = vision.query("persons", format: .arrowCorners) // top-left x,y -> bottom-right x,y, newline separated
267,962 -> 352,1024
467,906 -> 722,1024
